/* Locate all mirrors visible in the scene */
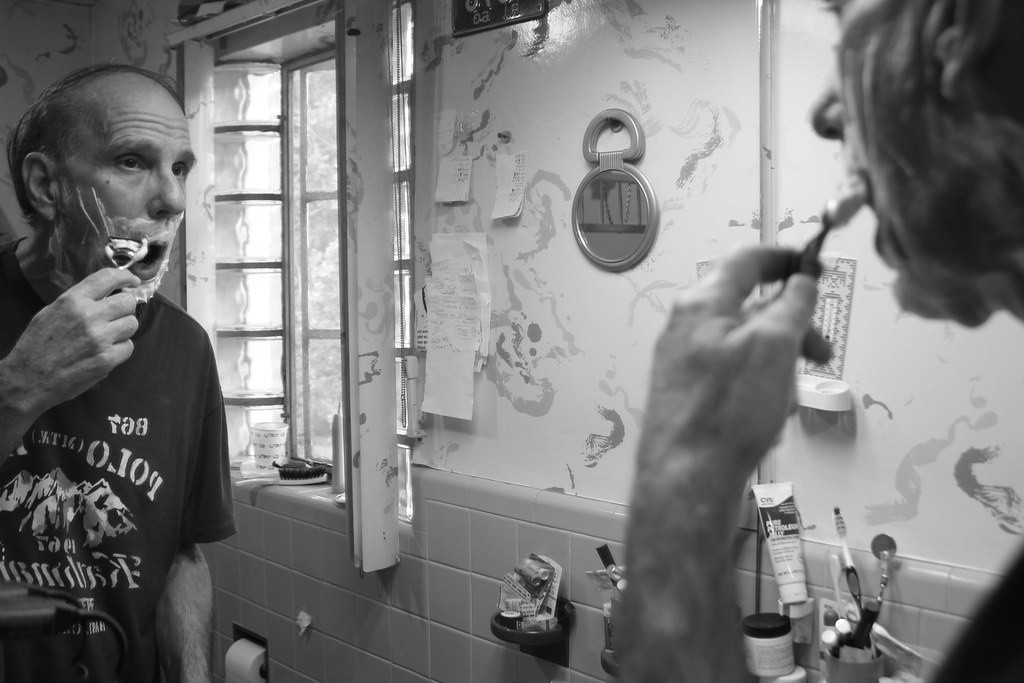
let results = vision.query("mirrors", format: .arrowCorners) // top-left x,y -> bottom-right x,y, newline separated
0,0 -> 781,682
571,107 -> 660,273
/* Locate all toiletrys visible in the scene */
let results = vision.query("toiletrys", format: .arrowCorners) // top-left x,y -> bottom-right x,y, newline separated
759,662 -> 807,683
751,482 -> 810,604
742,611 -> 797,678
818,600 -> 881,675
594,539 -> 629,660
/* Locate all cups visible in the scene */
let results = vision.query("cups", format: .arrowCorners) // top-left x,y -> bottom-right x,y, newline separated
248,421 -> 291,473
823,647 -> 886,683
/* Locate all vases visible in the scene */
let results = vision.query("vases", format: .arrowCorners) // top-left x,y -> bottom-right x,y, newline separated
250,421 -> 289,471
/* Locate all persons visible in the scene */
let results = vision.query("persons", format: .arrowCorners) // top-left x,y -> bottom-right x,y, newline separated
1,61 -> 241,683
600,1 -> 1024,682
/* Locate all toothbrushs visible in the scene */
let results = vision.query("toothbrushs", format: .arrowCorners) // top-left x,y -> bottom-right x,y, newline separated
327,378 -> 348,498
831,503 -> 878,656
869,620 -> 943,670
829,553 -> 852,629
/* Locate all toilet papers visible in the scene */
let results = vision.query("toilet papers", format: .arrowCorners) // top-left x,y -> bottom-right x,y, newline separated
220,636 -> 268,682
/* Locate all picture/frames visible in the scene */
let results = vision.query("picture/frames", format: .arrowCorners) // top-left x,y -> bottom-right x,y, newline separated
450,0 -> 546,37
